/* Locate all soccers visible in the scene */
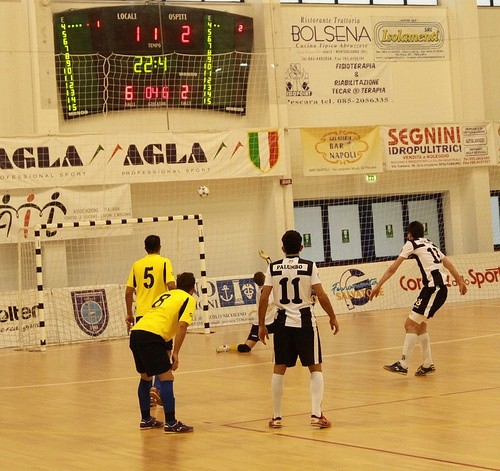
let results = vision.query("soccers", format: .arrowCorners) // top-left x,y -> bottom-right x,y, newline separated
198,185 -> 209,198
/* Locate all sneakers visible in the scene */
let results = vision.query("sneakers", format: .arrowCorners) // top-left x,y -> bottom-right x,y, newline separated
140,417 -> 163,430
415,363 -> 436,376
383,361 -> 408,376
310,411 -> 332,428
268,417 -> 282,428
164,420 -> 194,434
216,344 -> 229,353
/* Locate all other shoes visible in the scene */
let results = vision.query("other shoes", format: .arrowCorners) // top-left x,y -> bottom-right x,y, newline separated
150,386 -> 161,407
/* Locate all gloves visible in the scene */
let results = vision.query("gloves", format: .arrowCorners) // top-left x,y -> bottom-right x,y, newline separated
258,249 -> 269,260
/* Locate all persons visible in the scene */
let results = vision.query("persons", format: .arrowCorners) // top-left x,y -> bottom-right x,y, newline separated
125,235 -> 175,407
368,221 -> 467,376
258,230 -> 339,428
216,249 -> 274,353
129,273 -> 196,434
